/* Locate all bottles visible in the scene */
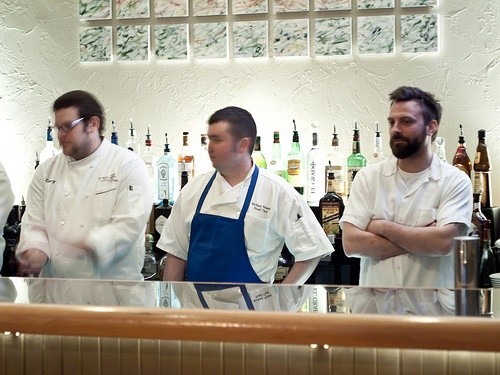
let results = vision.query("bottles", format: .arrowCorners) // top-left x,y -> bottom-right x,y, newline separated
251,136 -> 266,170
474,128 -> 493,208
306,132 -> 326,204
111,120 -> 197,205
325,125 -> 347,202
452,124 -> 472,179
471,193 -> 487,239
267,131 -> 287,181
319,160 -> 344,249
35,116 -> 62,171
366,121 -> 388,166
1,197 -> 26,276
286,130 -> 305,199
141,234 -> 168,280
347,122 -> 367,201
479,220 -> 496,289
196,133 -> 212,176
154,197 -> 173,256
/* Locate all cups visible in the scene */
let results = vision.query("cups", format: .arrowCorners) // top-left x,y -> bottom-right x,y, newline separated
489,273 -> 500,288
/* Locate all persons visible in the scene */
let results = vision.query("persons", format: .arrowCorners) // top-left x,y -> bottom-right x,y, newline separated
343,287 -> 456,316
156,106 -> 335,284
339,87 -> 473,288
172,283 -> 311,312
15,90 -> 154,281
24,278 -> 154,307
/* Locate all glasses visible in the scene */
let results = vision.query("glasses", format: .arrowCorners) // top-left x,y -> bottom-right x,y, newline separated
54,118 -> 85,133
